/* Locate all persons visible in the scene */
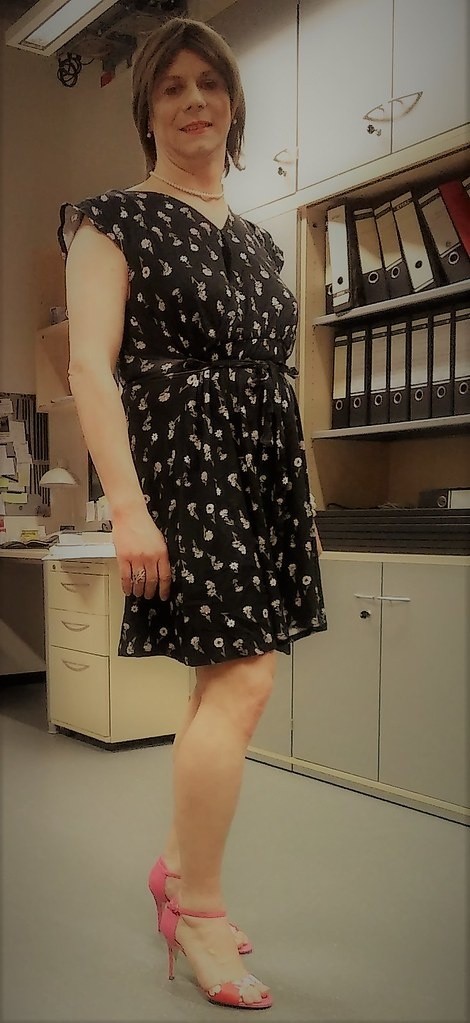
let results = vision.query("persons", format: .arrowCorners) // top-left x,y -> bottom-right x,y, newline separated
58,16 -> 333,1007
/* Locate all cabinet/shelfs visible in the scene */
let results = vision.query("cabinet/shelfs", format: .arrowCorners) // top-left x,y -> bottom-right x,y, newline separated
0,2 -> 470,822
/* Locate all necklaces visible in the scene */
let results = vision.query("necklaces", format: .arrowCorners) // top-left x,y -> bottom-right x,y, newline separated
149,170 -> 224,201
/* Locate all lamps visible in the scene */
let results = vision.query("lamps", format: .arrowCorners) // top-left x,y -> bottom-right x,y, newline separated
30,458 -> 79,488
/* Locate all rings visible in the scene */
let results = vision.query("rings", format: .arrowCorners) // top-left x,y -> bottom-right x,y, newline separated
132,567 -> 145,585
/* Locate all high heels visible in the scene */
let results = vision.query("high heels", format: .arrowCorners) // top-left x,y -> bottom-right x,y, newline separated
148,856 -> 252,955
161,896 -> 273,1009
